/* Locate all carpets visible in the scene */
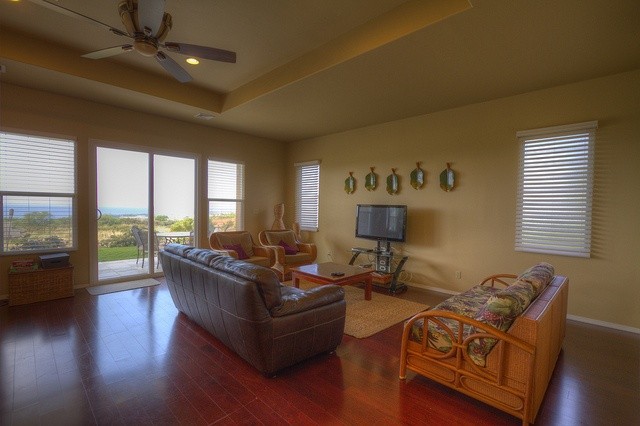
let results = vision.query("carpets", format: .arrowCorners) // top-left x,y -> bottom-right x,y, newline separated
280,278 -> 430,338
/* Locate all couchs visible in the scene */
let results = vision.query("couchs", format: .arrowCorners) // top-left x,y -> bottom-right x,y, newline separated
210,232 -> 283,284
397,262 -> 569,426
159,242 -> 347,378
258,227 -> 319,281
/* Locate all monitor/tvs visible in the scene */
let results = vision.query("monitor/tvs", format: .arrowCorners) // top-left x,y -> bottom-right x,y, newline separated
352,205 -> 408,256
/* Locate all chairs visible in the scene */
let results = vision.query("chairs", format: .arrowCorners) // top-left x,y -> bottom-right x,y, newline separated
138,228 -> 160,269
130,225 -> 142,268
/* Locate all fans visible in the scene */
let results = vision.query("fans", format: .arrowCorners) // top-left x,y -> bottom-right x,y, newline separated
28,0 -> 236,83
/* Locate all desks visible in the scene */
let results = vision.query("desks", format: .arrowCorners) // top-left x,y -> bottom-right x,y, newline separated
154,232 -> 190,245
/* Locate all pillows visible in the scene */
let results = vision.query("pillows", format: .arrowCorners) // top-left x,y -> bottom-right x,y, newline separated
215,233 -> 254,260
266,232 -> 299,254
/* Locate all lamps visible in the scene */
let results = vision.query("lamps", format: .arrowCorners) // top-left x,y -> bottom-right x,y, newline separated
117,1 -> 174,56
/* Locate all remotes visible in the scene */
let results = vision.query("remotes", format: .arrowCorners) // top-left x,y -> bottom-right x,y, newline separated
331,271 -> 345,276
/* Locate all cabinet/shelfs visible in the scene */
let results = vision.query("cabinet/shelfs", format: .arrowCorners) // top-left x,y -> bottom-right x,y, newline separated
350,247 -> 409,295
